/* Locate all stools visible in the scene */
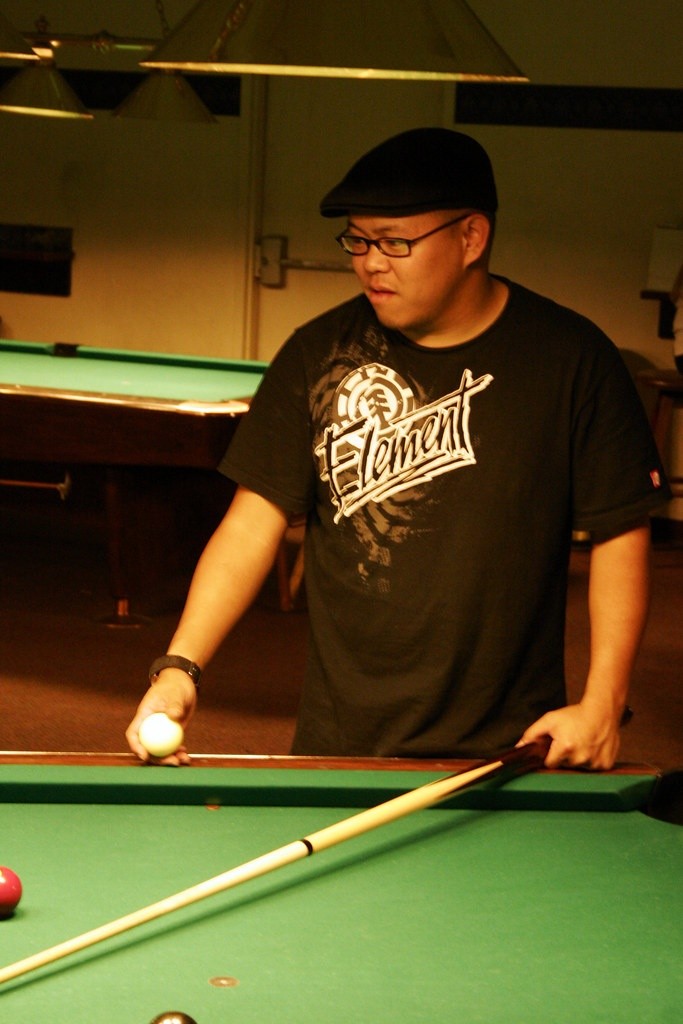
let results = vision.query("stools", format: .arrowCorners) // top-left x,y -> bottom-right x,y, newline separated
636,369 -> 683,500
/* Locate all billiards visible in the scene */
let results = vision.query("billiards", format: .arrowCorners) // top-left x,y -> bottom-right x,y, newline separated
151,1010 -> 197,1024
0,864 -> 23,915
138,712 -> 185,758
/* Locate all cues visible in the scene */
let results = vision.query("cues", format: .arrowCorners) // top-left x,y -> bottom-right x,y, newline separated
0,700 -> 639,987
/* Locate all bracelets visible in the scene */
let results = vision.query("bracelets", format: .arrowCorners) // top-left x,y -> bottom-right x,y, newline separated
148,655 -> 201,697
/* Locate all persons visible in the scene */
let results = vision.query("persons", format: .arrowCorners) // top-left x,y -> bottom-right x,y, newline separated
125,126 -> 670,774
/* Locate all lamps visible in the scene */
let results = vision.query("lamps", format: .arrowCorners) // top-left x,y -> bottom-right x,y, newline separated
0,0 -> 528,123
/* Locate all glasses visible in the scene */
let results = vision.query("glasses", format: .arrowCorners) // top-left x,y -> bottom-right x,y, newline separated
334,215 -> 469,255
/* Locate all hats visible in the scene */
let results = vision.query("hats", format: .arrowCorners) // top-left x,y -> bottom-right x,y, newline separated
319,128 -> 498,218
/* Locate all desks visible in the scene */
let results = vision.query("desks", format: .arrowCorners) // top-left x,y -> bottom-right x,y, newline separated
0,750 -> 683,1024
0,343 -> 271,628
